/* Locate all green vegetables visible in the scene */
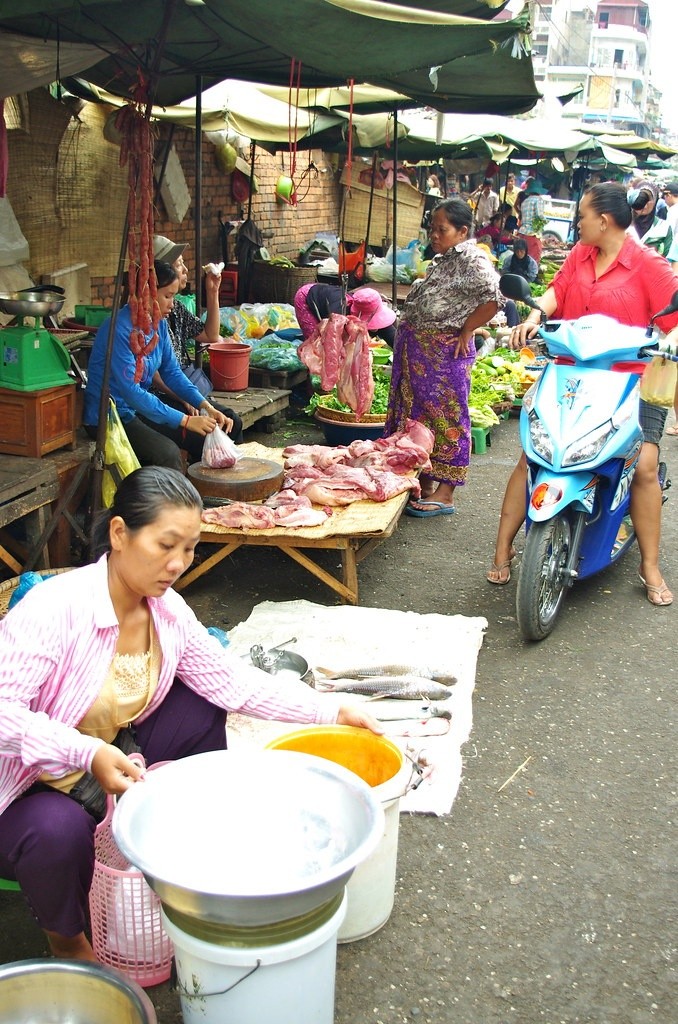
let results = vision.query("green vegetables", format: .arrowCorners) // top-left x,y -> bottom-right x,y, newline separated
188,324 -> 301,372
301,365 -> 391,416
488,348 -> 521,363
531,217 -> 548,232
467,370 -> 524,427
530,258 -> 560,297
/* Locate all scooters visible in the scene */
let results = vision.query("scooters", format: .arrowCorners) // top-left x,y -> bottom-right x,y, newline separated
498,272 -> 678,643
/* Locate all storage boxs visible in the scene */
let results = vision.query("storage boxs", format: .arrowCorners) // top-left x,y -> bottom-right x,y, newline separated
0,385 -> 77,457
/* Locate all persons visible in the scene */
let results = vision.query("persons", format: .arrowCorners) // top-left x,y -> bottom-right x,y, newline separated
626,179 -> 678,274
427,174 -> 441,197
350,288 -> 397,349
135,235 -> 242,465
498,173 -> 524,219
294,283 -> 354,340
469,180 -> 500,227
83,259 -> 233,475
502,239 -> 539,283
514,178 -> 535,221
382,197 -> 502,517
0,465 -> 383,964
487,183 -> 678,606
518,180 -> 549,235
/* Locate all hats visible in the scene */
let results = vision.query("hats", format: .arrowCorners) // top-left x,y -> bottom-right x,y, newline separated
524,180 -> 549,194
661,183 -> 678,199
351,288 -> 396,329
153,235 -> 190,266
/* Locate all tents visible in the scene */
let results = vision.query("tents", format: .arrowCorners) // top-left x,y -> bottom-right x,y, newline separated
0,0 -> 678,181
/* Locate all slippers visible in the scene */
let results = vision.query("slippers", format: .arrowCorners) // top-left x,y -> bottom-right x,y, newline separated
488,554 -> 517,584
666,425 -> 678,435
406,498 -> 454,517
638,573 -> 673,606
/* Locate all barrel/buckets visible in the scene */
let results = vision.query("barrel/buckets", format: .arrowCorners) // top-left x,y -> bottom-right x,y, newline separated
207,343 -> 253,392
159,880 -> 348,1024
262,725 -> 425,946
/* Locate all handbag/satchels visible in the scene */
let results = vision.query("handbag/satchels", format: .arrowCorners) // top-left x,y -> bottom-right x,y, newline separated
181,362 -> 213,398
200,408 -> 245,468
337,242 -> 365,283
386,240 -> 422,273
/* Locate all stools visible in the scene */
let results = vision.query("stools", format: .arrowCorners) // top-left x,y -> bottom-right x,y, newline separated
219,270 -> 238,308
471,426 -> 491,455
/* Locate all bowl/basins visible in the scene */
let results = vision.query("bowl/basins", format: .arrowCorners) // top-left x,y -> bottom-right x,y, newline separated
313,411 -> 385,447
111,747 -> 386,927
369,348 -> 391,365
0,956 -> 158,1024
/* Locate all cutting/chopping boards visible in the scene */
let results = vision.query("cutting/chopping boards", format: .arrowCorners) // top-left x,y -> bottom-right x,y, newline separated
186,456 -> 284,502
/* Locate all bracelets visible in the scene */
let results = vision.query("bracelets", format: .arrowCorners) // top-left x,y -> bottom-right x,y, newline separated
182,415 -> 190,438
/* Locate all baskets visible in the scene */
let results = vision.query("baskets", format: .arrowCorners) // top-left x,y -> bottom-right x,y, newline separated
253,258 -> 317,307
89,754 -> 177,986
315,394 -> 387,423
0,567 -> 79,621
525,356 -> 553,371
